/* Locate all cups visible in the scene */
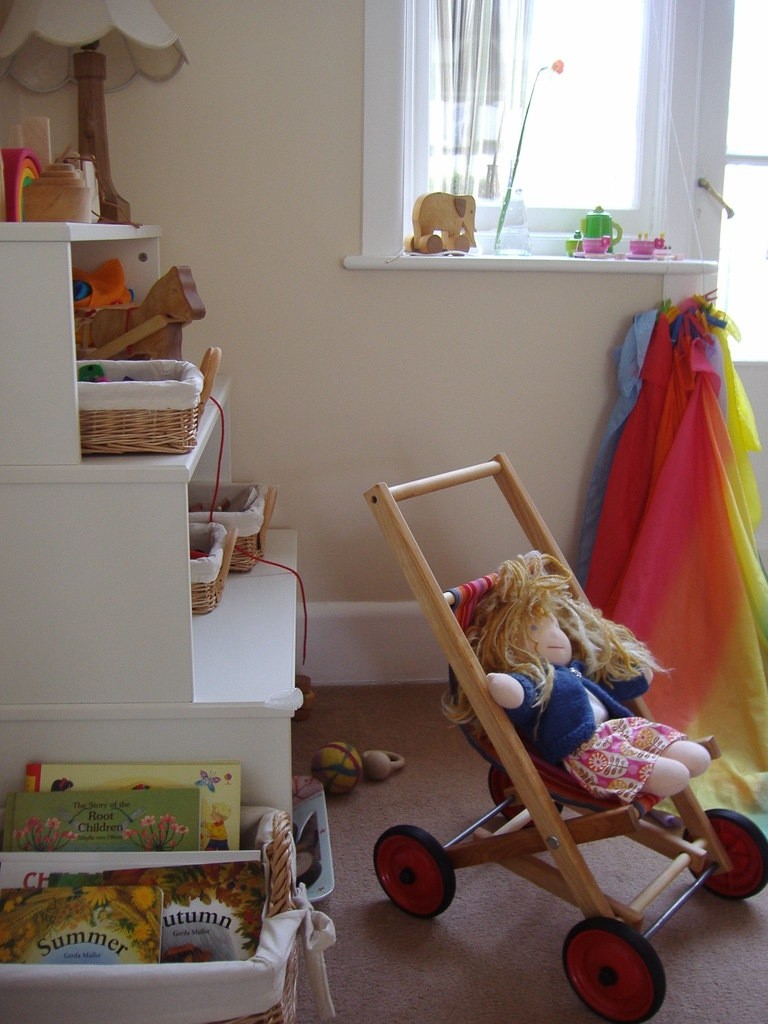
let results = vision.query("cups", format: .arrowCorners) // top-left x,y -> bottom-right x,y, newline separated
564,239 -> 582,257
582,236 -> 610,254
629,240 -> 654,254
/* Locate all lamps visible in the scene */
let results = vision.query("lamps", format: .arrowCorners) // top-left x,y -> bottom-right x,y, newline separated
0,0 -> 190,227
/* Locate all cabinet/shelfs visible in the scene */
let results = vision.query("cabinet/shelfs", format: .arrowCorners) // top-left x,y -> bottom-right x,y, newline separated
0,222 -> 304,826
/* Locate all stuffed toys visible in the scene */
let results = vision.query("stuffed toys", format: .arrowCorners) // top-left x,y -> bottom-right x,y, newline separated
442,552 -> 711,797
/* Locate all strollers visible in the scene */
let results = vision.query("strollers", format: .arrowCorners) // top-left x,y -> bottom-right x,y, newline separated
373,452 -> 768,1024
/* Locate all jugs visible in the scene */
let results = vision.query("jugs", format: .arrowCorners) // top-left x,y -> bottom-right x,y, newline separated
581,206 -> 623,251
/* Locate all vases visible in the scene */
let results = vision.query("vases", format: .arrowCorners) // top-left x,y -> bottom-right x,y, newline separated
493,187 -> 532,256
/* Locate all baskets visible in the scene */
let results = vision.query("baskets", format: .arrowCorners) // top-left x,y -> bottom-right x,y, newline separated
0,804 -> 297,1024
187,478 -> 277,614
76,346 -> 222,454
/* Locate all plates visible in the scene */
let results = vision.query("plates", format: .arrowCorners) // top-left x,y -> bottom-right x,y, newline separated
626,252 -> 653,259
573,251 -> 585,259
583,253 -> 612,259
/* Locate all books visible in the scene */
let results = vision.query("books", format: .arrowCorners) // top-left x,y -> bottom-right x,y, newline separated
1,763 -> 265,963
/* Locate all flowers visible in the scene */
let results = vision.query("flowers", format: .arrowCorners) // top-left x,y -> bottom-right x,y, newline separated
491,59 -> 566,250
